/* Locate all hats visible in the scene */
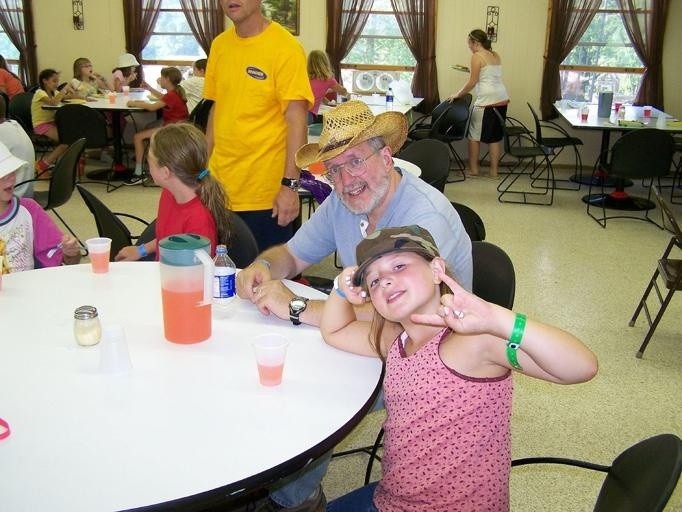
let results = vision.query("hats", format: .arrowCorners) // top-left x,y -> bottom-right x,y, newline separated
0,141 -> 28,179
353,225 -> 440,287
115,54 -> 140,68
295,101 -> 409,169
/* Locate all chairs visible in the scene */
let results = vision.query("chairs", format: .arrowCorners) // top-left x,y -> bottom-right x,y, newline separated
588,128 -> 676,228
367,242 -> 515,485
642,132 -> 682,205
13,137 -> 89,257
493,109 -> 557,205
526,102 -> 584,190
134,208 -> 259,269
142,99 -> 214,188
510,426 -> 682,511
629,185 -> 682,358
393,140 -> 450,194
76,182 -> 150,262
409,92 -> 472,183
10,83 -> 63,179
57,82 -> 68,91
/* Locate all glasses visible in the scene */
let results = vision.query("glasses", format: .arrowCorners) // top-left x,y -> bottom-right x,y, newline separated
320,147 -> 385,185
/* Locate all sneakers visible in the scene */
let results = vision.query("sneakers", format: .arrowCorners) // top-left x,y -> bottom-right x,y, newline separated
124,173 -> 148,186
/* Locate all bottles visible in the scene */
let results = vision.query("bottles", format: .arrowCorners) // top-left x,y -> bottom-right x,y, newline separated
617,106 -> 625,120
212,245 -> 236,305
74,306 -> 101,347
385,88 -> 393,112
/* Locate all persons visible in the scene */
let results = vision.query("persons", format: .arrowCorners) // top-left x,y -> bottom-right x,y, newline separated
63,58 -> 118,164
204,0 -> 313,253
158,58 -> 208,114
0,56 -> 24,106
107,54 -> 140,91
236,101 -> 471,324
0,143 -> 82,273
126,68 -> 189,185
112,125 -> 230,262
307,50 -> 351,124
1,93 -> 35,198
318,227 -> 597,512
448,30 -> 510,179
31,69 -> 73,178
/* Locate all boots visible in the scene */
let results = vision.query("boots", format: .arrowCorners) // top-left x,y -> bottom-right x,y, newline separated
35,160 -> 49,178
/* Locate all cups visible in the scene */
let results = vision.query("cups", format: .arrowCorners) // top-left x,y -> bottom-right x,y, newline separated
108,91 -> 117,105
86,237 -> 112,274
615,102 -> 623,113
158,232 -> 215,346
580,107 -> 589,123
643,106 -> 652,118
122,85 -> 130,96
254,341 -> 287,388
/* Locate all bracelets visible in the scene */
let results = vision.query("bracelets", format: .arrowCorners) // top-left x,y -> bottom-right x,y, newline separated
62,88 -> 68,95
255,257 -> 272,269
135,241 -> 148,258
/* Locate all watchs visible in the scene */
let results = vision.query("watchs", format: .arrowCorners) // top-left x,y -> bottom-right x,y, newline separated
506,314 -> 526,369
288,296 -> 308,325
280,176 -> 301,190
332,273 -> 348,301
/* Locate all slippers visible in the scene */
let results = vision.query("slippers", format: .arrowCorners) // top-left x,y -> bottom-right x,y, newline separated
482,174 -> 502,181
456,168 -> 481,179
